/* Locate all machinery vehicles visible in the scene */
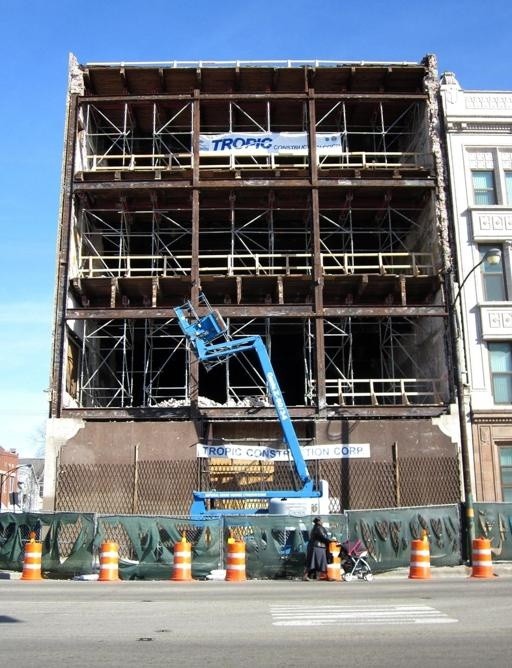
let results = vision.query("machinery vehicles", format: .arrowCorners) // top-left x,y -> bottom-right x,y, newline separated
172,292 -> 330,560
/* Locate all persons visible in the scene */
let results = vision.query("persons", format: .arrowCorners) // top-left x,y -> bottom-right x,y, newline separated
302,515 -> 338,581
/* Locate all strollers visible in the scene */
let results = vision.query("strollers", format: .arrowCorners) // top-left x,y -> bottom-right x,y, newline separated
334,538 -> 374,583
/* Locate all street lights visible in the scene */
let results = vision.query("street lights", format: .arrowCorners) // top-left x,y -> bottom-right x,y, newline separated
440,248 -> 501,565
0,463 -> 32,513
